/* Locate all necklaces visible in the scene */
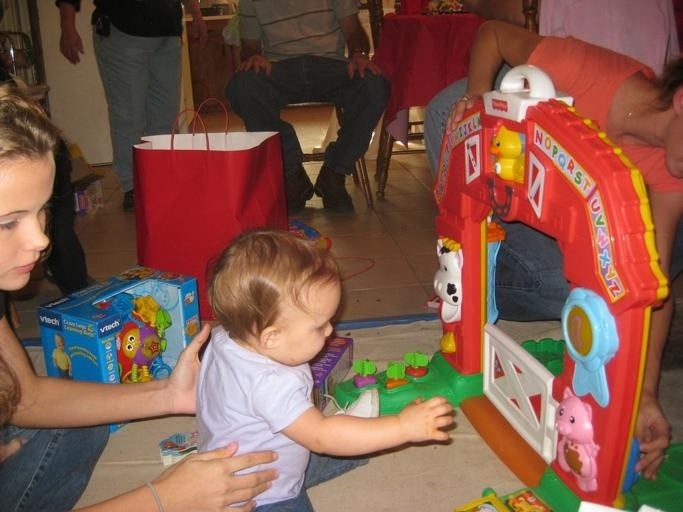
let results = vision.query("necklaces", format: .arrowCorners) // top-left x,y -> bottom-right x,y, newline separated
619,99 -> 644,136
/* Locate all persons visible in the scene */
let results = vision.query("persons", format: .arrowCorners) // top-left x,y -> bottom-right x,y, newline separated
224,0 -> 391,214
52,1 -> 210,213
0,140 -> 86,331
0,67 -> 280,511
462,0 -> 680,81
192,225 -> 458,511
420,18 -> 683,483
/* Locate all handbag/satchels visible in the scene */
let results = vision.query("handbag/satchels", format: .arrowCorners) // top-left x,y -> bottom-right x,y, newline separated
131,130 -> 290,322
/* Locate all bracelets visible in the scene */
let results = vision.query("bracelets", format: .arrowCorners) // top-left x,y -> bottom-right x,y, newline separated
349,49 -> 369,57
145,478 -> 165,511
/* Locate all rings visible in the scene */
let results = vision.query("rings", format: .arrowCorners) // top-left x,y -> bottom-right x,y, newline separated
461,96 -> 471,101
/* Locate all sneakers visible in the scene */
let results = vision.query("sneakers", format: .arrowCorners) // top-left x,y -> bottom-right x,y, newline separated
287,177 -> 314,213
333,388 -> 380,419
314,163 -> 355,214
123,190 -> 135,212
44,260 -> 96,296
